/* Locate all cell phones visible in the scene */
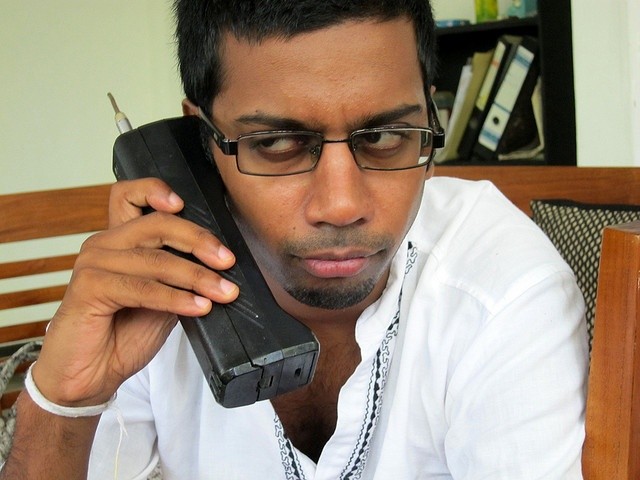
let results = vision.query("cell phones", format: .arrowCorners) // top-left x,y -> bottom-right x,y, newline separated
106,89 -> 322,409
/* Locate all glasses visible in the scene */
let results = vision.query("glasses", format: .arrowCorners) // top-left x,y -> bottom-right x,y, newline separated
199,83 -> 445,175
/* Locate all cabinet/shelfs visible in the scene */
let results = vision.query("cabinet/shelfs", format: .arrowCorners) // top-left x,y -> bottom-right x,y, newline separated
429,0 -> 578,166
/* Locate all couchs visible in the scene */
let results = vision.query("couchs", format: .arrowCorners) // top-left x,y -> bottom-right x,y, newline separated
0,164 -> 640,479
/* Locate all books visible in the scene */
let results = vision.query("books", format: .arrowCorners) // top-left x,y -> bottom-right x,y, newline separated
431,34 -> 545,165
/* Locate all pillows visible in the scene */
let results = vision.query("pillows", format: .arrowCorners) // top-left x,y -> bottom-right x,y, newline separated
528,197 -> 639,376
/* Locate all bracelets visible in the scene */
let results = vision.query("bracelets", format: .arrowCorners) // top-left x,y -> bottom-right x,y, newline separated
23,358 -> 118,418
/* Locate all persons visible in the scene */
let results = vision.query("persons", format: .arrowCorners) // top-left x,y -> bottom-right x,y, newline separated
1,0 -> 590,480
